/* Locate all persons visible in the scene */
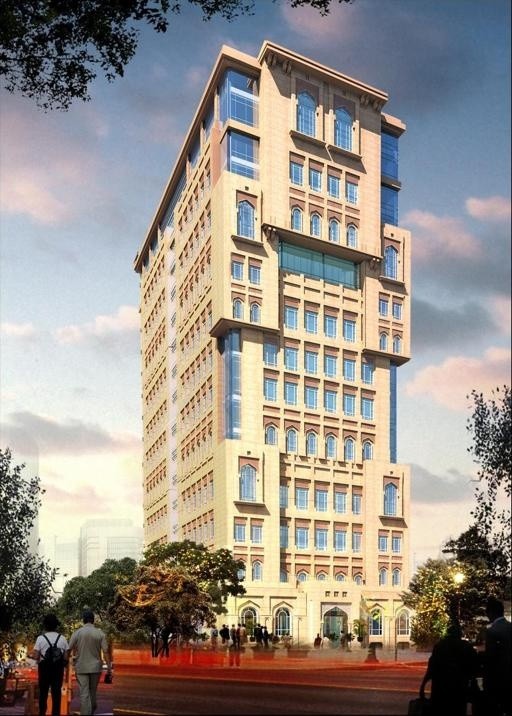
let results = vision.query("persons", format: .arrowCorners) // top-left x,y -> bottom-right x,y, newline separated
340,630 -> 346,650
210,623 -> 269,650
314,633 -> 321,650
482,597 -> 512,716
66,611 -> 112,716
419,626 -> 479,716
32,614 -> 69,716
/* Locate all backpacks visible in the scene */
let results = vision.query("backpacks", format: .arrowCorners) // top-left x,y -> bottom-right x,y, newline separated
39,634 -> 63,671
